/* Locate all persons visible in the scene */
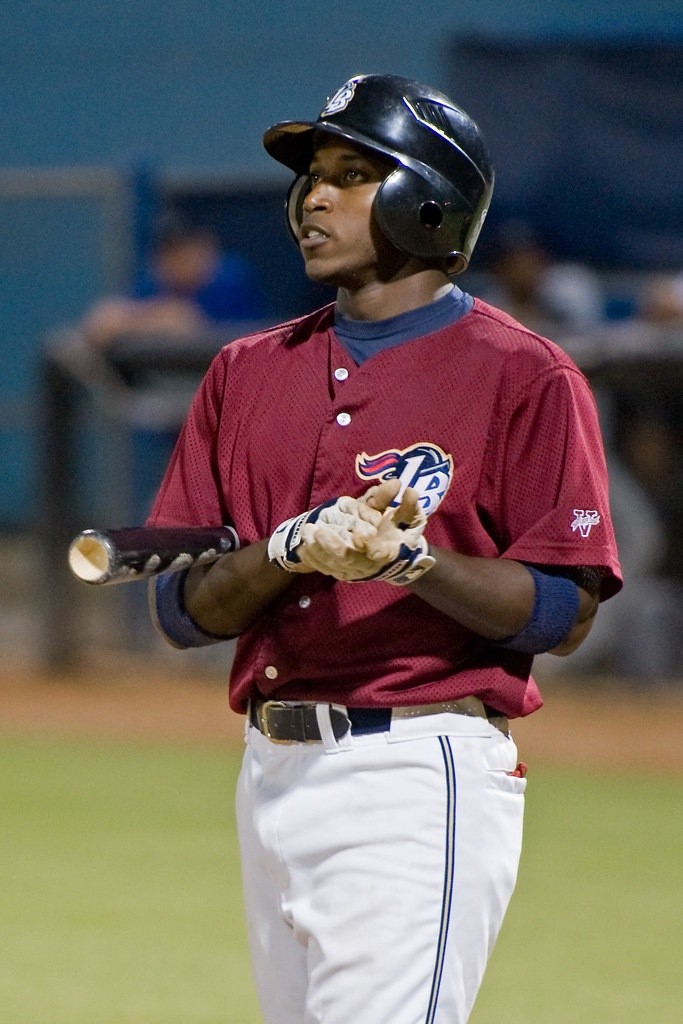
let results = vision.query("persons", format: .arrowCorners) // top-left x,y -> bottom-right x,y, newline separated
142,70 -> 626,1024
459,216 -> 683,682
75,209 -> 257,667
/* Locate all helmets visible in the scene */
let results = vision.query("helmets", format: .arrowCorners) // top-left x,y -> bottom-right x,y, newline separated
260,72 -> 496,277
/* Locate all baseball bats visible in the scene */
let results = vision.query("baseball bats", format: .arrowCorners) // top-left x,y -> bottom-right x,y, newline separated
62,523 -> 243,590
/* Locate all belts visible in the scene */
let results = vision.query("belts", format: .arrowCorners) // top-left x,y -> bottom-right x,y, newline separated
247,694 -> 510,745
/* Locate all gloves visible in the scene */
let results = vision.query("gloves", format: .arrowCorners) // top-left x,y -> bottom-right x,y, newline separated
297,486 -> 436,587
267,477 -> 403,575
